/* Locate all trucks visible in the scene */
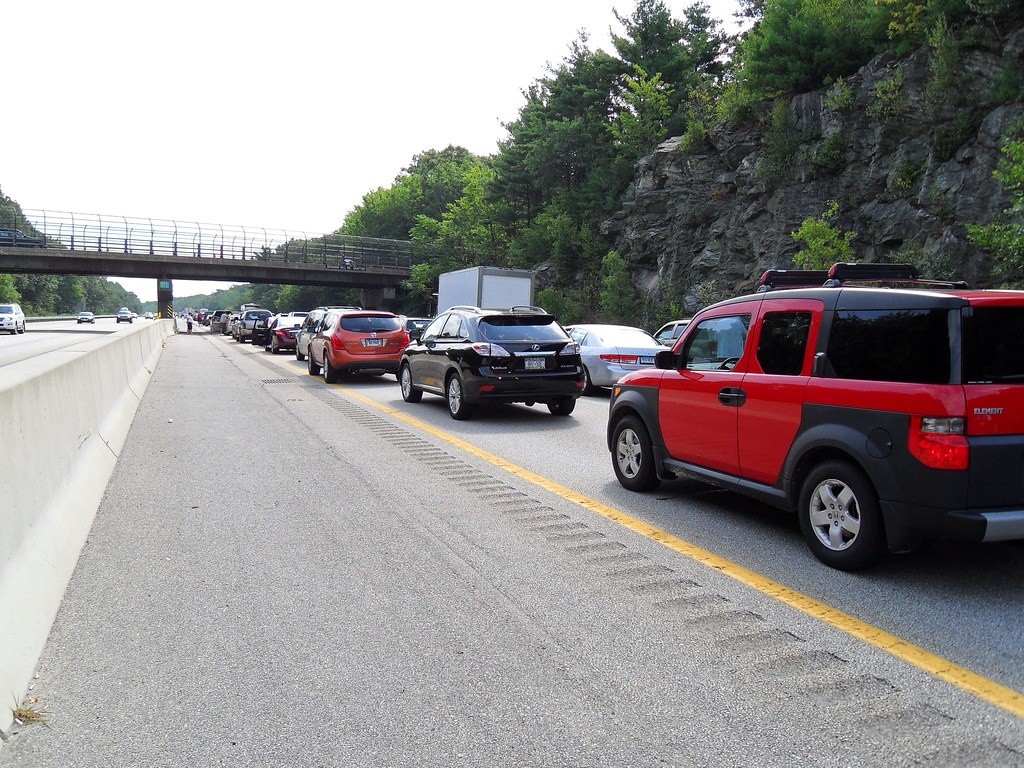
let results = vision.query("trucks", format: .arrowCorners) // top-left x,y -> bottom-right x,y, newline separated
430,266 -> 536,322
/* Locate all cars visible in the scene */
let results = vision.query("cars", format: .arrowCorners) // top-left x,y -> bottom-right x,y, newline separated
190,303 -> 309,345
132,313 -> 138,319
564,324 -> 674,397
0,228 -> 44,249
144,311 -> 159,319
78,311 -> 95,325
405,316 -> 436,344
264,316 -> 306,354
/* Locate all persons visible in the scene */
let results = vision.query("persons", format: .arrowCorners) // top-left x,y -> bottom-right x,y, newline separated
714,318 -> 747,361
187,312 -> 193,334
220,312 -> 230,336
198,312 -> 203,327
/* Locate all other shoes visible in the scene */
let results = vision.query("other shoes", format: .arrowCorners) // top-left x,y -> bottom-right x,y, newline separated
220,333 -> 224,336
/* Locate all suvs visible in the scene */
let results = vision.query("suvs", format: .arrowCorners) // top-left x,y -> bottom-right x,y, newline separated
306,308 -> 410,384
606,260 -> 1023,572
0,303 -> 26,335
651,318 -> 724,368
116,311 -> 133,324
294,305 -> 364,363
398,306 -> 585,420
